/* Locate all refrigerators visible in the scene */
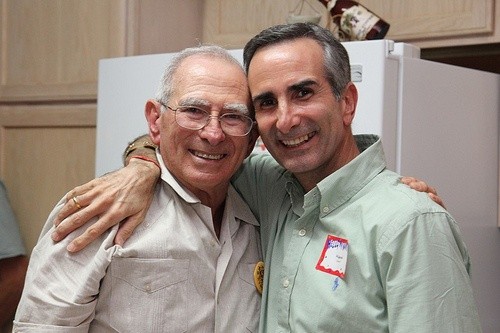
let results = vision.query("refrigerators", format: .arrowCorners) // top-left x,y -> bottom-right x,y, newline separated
95,41 -> 500,333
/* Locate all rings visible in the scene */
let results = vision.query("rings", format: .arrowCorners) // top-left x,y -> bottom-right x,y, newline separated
73,197 -> 83,210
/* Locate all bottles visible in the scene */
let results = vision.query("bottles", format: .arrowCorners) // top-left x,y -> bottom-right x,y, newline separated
318,0 -> 390,40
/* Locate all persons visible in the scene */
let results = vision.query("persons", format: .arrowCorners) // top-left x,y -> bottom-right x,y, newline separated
52,22 -> 482,332
11,46 -> 448,332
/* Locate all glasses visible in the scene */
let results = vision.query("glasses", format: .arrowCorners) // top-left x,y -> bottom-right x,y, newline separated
157,97 -> 258,138
